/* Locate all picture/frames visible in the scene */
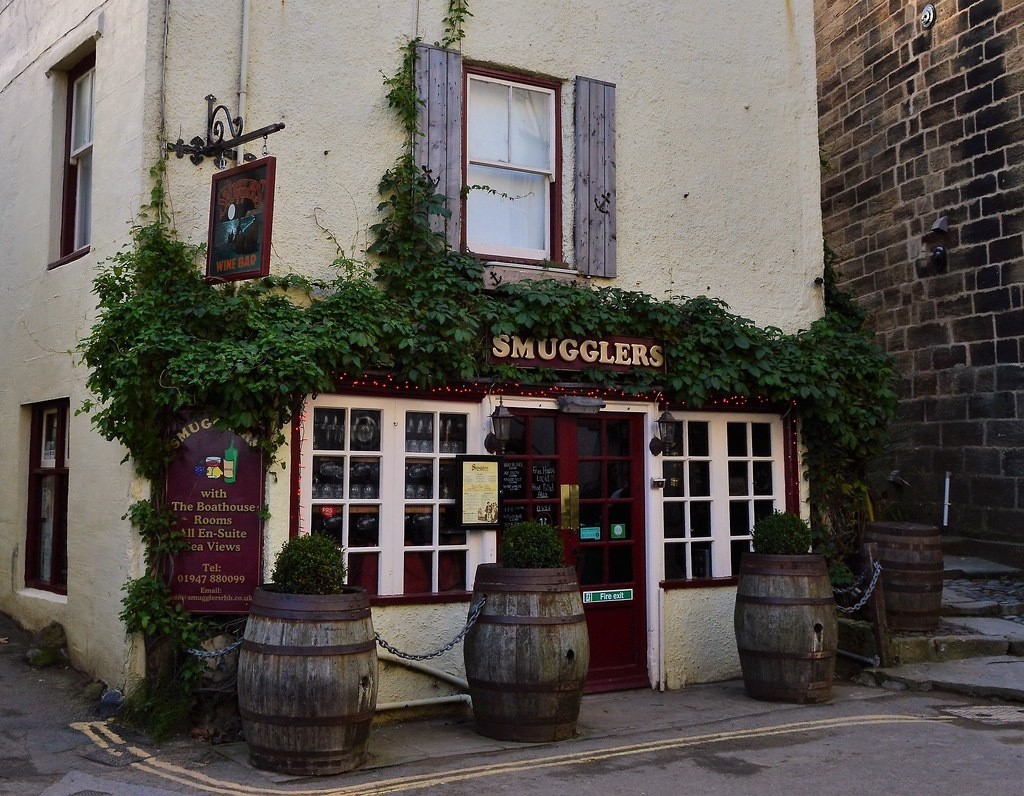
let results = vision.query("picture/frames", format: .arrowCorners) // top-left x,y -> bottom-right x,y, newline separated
205,155 -> 277,284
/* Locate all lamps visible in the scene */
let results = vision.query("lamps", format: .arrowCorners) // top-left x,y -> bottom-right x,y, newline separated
558,395 -> 607,415
484,395 -> 514,456
650,407 -> 677,455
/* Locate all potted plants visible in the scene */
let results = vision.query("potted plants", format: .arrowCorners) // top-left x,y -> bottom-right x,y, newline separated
463,522 -> 590,742
734,510 -> 838,704
237,529 -> 378,776
